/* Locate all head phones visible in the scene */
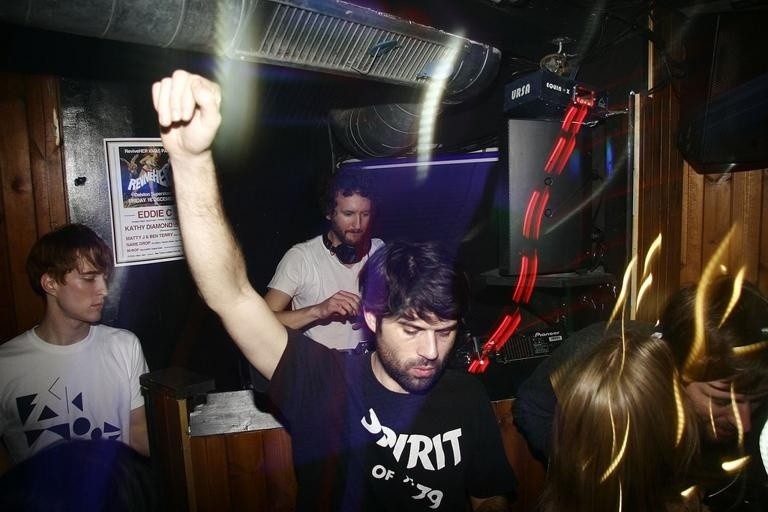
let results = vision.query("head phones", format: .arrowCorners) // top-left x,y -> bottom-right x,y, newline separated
323,222 -> 371,264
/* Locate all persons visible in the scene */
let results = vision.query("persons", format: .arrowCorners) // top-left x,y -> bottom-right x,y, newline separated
1,223 -> 155,464
511,272 -> 768,511
150,70 -> 518,511
263,173 -> 392,356
532,328 -> 711,512
